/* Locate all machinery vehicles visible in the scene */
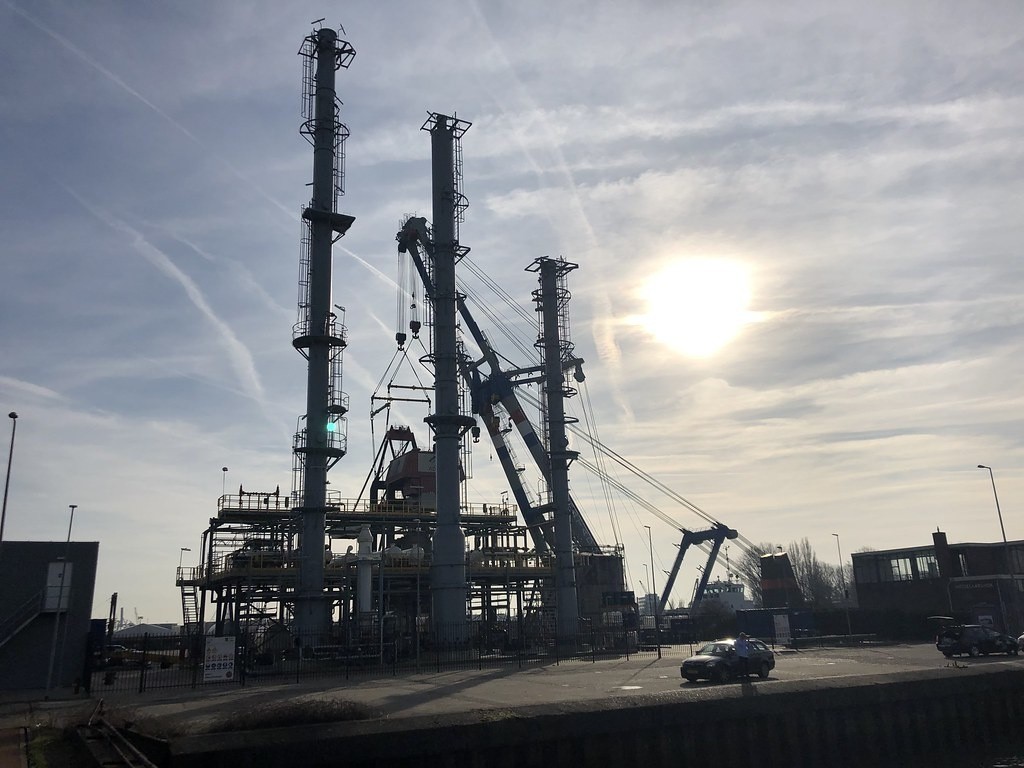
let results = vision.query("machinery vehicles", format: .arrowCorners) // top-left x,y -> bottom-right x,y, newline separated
373,213 -> 639,657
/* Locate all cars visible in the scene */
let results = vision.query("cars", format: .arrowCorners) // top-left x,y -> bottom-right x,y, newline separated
680,638 -> 775,685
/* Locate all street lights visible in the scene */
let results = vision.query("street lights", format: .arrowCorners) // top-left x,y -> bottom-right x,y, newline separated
644,526 -> 661,658
978,465 -> 1014,583
0,412 -> 18,541
832,534 -> 851,635
44,505 -> 78,702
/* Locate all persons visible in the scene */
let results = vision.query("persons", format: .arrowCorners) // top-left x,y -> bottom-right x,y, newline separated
734,632 -> 750,682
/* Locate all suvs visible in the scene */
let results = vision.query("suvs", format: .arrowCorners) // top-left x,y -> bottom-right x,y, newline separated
927,616 -> 1024,659
